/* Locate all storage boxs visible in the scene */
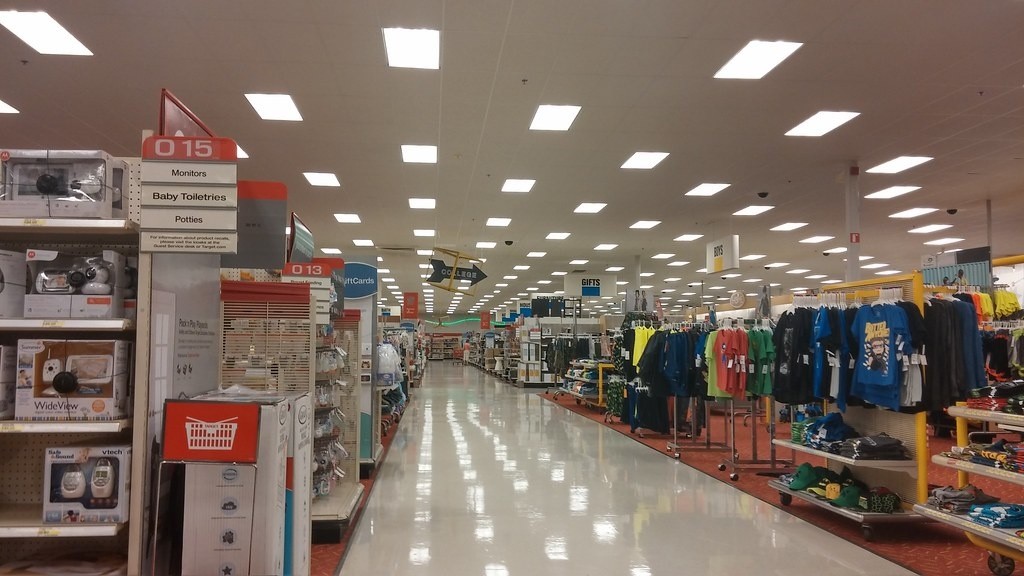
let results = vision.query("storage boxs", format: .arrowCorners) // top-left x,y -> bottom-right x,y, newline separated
544,374 -> 559,382
520,325 -> 528,341
535,365 -> 539,382
523,343 -> 529,361
518,363 -> 523,380
520,364 -> 526,381
42,437 -> 133,524
535,345 -> 539,361
0,547 -> 128,576
542,351 -> 546,358
529,364 -> 534,382
124,256 -> 138,318
24,248 -> 126,319
542,361 -> 547,369
0,249 -> 26,317
526,363 -> 529,381
126,342 -> 135,418
0,345 -> 17,382
0,149 -> 114,218
14,339 -> 130,421
0,383 -> 15,420
530,344 -> 536,361
112,159 -> 130,220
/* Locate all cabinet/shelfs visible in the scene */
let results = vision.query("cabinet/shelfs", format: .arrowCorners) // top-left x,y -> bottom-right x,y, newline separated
913,394 -> 1024,576
560,362 -> 615,415
0,130 -> 425,576
912,402 -> 1024,576
516,315 -> 602,388
425,333 -> 463,361
767,272 -> 923,541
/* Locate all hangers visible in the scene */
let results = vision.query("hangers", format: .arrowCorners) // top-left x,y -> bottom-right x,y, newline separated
557,284 -> 1024,393
